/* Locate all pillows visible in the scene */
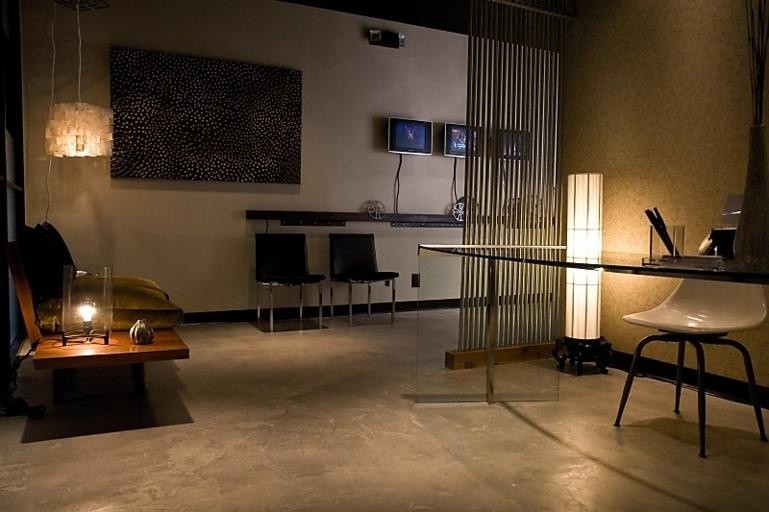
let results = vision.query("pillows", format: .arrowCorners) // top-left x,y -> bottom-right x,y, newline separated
20,221 -> 76,301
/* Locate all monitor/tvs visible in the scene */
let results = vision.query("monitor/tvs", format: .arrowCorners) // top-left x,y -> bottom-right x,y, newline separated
443,122 -> 482,159
387,117 -> 433,155
497,128 -> 532,163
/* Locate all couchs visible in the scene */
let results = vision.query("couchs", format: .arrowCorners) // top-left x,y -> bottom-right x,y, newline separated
7,241 -> 185,355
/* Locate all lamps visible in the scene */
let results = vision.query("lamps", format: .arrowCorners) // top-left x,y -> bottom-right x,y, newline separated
78,305 -> 94,334
44,0 -> 114,158
552,171 -> 613,376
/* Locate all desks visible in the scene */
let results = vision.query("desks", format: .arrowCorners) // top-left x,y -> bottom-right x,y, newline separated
415,247 -> 769,404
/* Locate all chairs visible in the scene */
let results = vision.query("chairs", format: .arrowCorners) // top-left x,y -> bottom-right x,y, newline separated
254,233 -> 326,333
614,278 -> 769,458
329,232 -> 399,327
